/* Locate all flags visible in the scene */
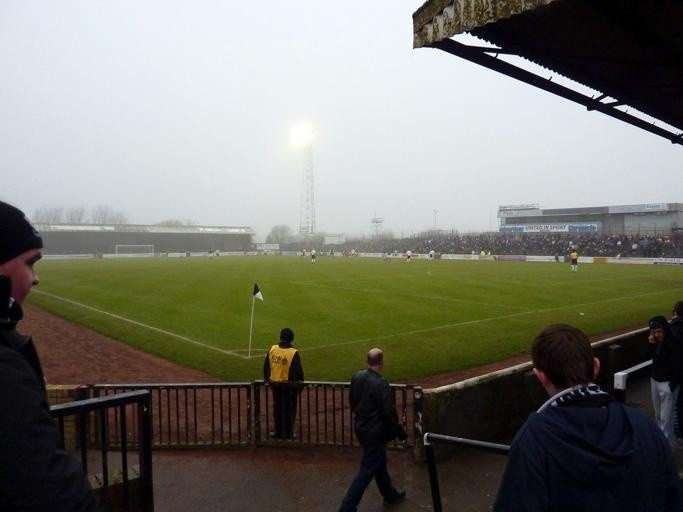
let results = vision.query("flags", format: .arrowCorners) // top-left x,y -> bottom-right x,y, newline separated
253,283 -> 264,302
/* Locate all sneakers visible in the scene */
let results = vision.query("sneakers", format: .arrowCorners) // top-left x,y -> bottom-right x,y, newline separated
286,433 -> 296,443
270,433 -> 282,443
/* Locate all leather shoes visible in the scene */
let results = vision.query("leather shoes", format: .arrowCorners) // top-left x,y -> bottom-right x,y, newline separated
383,489 -> 406,507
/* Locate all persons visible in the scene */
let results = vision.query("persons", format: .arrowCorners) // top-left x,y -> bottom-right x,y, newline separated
311,248 -> 316,264
317,227 -> 683,258
207,248 -> 436,263
570,251 -> 579,272
647,316 -> 680,451
489,321 -> 682,511
264,328 -> 304,440
667,300 -> 683,442
338,347 -> 406,511
0,198 -> 100,510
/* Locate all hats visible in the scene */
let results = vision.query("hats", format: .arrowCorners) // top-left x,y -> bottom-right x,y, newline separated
0,200 -> 44,267
